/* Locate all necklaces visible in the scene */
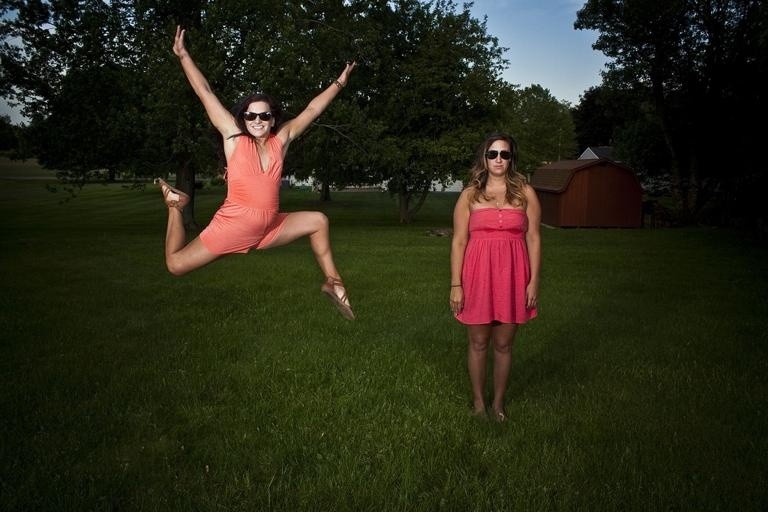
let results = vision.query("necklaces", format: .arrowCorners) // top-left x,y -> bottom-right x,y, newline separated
493,197 -> 503,208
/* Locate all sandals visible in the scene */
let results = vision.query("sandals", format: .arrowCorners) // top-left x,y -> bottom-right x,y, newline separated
157,178 -> 190,212
320,276 -> 355,323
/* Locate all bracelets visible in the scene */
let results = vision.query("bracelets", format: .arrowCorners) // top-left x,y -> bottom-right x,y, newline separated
180,53 -> 191,59
450,285 -> 463,288
333,79 -> 343,92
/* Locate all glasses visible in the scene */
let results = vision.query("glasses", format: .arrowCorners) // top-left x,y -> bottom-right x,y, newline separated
486,151 -> 512,160
243,112 -> 272,121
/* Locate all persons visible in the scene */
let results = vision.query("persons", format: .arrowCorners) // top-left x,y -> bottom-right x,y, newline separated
448,134 -> 542,422
157,25 -> 355,322
